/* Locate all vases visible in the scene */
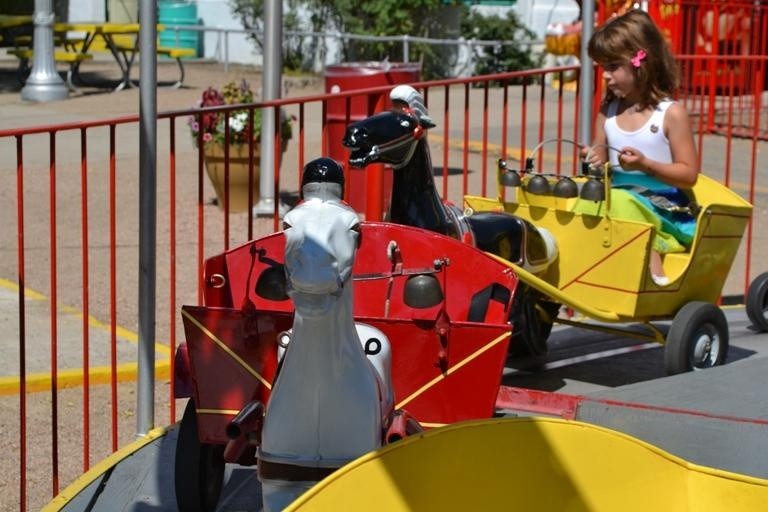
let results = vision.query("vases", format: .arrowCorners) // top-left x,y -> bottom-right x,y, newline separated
202,144 -> 259,213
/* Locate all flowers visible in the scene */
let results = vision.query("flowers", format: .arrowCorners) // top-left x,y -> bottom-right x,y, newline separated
186,76 -> 293,158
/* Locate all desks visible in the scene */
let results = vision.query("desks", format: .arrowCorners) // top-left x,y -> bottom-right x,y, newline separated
1,13 -> 167,96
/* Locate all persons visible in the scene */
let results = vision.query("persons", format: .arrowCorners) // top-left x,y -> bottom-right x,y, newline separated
575,9 -> 700,285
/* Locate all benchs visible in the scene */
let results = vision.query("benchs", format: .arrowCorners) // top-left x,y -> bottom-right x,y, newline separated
0,36 -> 197,96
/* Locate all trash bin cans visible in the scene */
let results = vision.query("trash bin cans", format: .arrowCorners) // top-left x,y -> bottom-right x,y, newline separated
322,58 -> 423,222
